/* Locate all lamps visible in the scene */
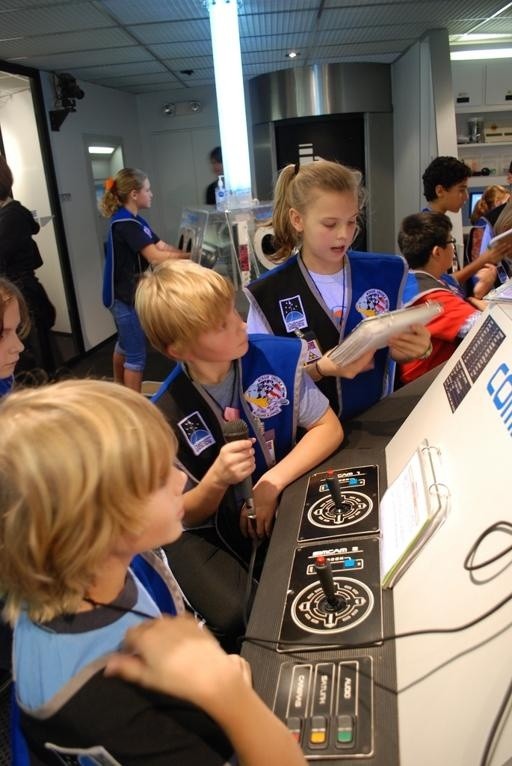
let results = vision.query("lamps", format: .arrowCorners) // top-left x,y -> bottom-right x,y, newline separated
157,98 -> 205,117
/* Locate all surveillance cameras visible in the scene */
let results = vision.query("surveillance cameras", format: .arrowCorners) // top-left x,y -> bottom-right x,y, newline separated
58,72 -> 84,100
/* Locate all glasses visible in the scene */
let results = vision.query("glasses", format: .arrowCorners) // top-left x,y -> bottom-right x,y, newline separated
445,237 -> 456,245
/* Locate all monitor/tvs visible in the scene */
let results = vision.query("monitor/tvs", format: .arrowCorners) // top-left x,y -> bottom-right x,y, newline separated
467,191 -> 486,218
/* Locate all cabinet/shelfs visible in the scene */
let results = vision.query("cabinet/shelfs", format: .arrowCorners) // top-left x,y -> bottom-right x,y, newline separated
482,56 -> 512,114
449,55 -> 485,115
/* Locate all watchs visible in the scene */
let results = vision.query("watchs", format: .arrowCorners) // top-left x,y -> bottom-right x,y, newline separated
420,343 -> 433,359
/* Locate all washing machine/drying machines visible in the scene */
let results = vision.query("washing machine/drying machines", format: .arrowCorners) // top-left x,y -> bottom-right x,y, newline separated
177,197 -> 293,282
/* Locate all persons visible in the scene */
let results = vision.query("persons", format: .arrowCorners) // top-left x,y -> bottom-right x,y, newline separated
422,156 -> 511,288
397,211 -> 488,383
2,377 -> 306,764
464,185 -> 510,300
244,159 -> 430,442
134,258 -> 344,588
1,158 -> 57,384
98,167 -> 191,394
206,148 -> 224,205
472,165 -> 512,257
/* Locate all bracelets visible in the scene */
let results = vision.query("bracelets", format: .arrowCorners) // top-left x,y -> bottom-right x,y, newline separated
316,360 -> 325,377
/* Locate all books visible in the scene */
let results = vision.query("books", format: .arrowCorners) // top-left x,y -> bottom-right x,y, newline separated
327,301 -> 444,368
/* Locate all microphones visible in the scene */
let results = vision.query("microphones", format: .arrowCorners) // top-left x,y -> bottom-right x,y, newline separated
222,419 -> 256,519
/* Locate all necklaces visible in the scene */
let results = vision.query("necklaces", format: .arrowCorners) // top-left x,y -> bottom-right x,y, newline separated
193,361 -> 239,422
300,245 -> 345,324
82,594 -> 156,620
